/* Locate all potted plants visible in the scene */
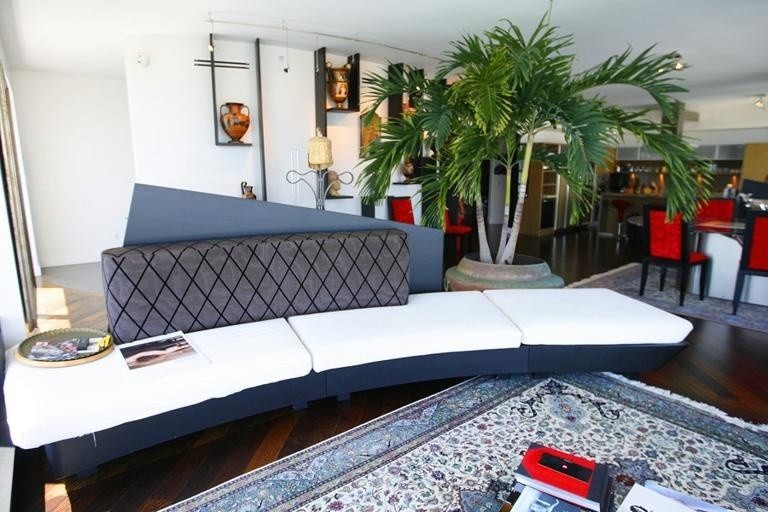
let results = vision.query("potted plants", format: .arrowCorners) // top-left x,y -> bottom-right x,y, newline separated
351,1 -> 722,290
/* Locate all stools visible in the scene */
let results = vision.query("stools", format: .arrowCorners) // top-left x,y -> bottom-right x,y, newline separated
611,198 -> 633,241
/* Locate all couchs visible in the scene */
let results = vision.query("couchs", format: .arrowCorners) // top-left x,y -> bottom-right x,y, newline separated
0,228 -> 697,484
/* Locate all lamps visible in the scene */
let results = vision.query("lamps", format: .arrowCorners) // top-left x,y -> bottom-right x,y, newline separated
754,93 -> 767,109
285,134 -> 354,210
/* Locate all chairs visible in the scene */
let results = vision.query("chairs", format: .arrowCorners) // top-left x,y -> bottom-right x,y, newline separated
636,195 -> 768,312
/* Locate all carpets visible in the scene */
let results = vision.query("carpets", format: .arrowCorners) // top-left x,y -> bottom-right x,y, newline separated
155,374 -> 768,511
564,260 -> 768,334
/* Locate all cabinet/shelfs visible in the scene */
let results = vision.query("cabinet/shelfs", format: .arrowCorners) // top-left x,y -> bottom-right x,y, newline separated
313,45 -> 450,229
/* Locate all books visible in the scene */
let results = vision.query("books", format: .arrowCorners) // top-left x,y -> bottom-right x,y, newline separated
115,329 -> 197,372
506,441 -> 614,512
